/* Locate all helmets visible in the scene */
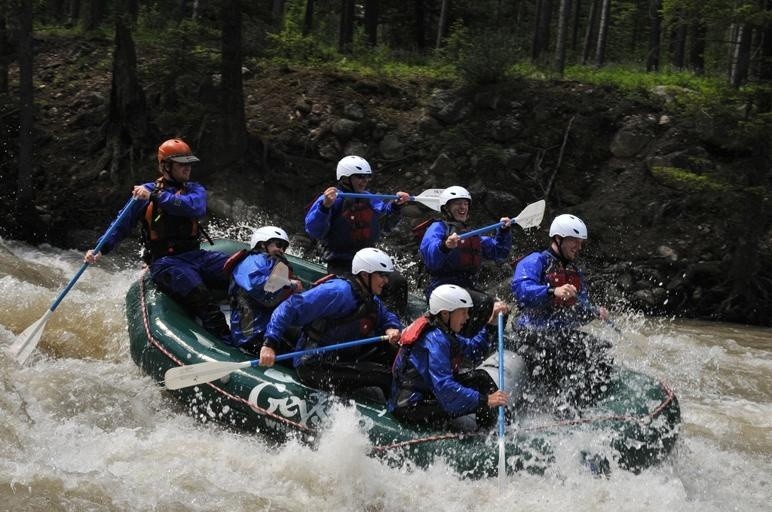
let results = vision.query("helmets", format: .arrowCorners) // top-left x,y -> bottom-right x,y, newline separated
429,284 -> 474,315
438,186 -> 473,214
158,138 -> 192,161
549,214 -> 588,240
351,247 -> 394,275
251,225 -> 290,249
336,156 -> 373,180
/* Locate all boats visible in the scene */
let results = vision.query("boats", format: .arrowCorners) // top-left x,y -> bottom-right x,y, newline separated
124,232 -> 683,477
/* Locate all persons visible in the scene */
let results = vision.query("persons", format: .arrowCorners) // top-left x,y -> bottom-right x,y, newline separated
509,214 -> 620,404
303,154 -> 411,283
417,186 -> 514,342
229,226 -> 315,372
387,283 -> 515,436
256,246 -> 406,405
84,139 -> 241,347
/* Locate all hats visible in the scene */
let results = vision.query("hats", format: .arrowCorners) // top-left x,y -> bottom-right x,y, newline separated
170,155 -> 199,164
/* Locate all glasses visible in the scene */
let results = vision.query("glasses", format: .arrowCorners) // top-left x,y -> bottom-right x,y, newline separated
355,172 -> 371,180
275,240 -> 288,251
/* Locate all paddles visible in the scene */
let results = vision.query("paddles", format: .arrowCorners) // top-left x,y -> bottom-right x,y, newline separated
332,186 -> 449,214
458,199 -> 547,242
9,196 -> 150,366
163,333 -> 399,392
264,261 -> 298,296
495,306 -> 508,493
